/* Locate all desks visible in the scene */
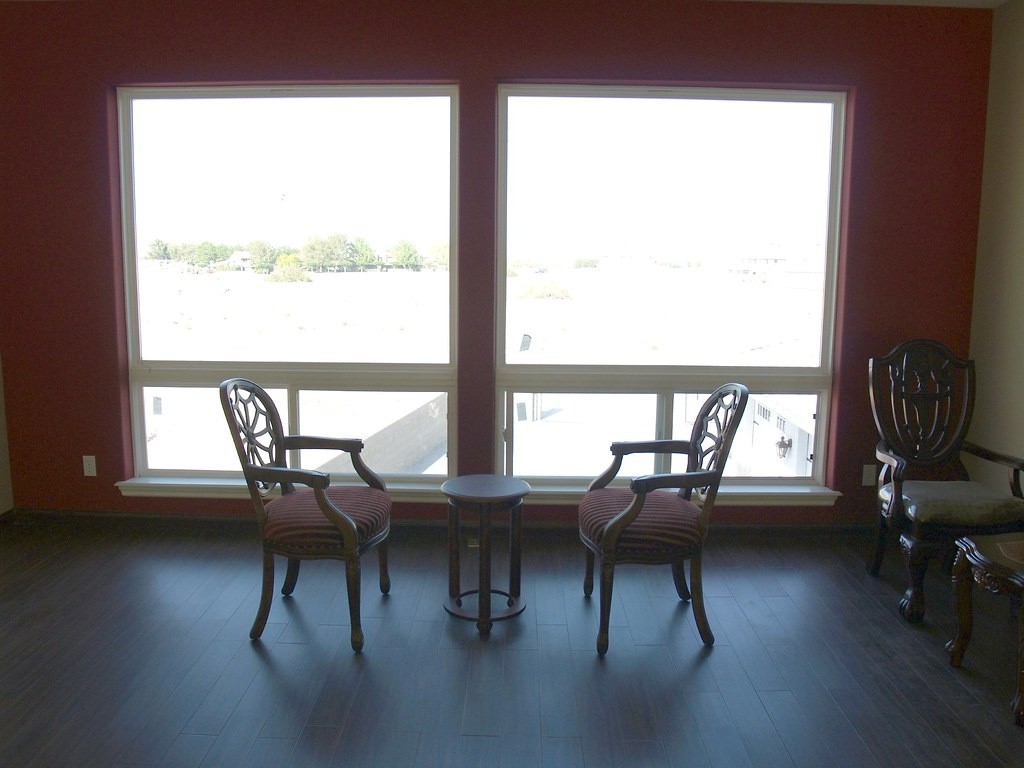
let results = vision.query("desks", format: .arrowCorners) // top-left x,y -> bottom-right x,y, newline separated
440,472 -> 533,636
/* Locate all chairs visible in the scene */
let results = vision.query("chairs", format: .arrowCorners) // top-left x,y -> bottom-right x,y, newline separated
220,378 -> 393,656
576,382 -> 750,657
866,336 -> 1024,627
942,530 -> 1024,730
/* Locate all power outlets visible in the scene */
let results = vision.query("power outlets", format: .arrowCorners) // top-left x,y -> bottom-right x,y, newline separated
82,455 -> 97,477
862,464 -> 877,488
